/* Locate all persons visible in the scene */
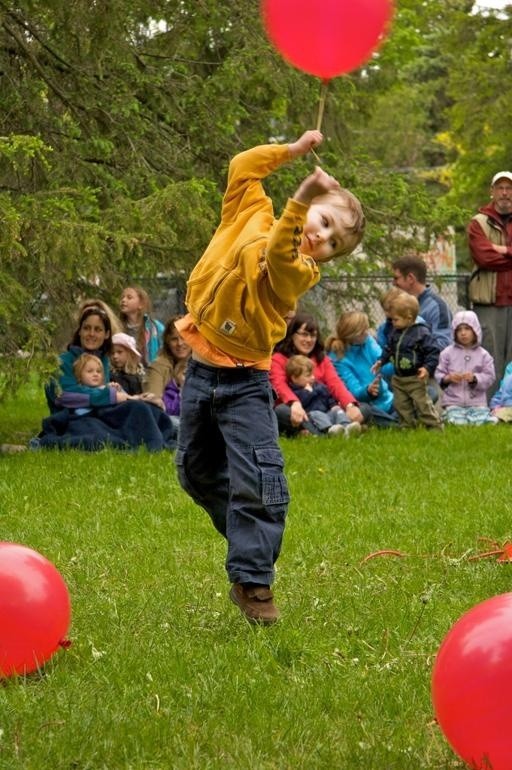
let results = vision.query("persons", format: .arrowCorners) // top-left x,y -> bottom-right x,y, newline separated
173,130 -> 366,624
270,313 -> 363,437
381,288 -> 425,348
80,299 -> 125,336
110,332 -> 142,395
73,354 -> 131,413
469,171 -> 512,406
393,255 -> 454,403
370,293 -> 444,429
145,316 -> 194,396
32,310 -> 179,452
329,311 -> 403,425
164,358 -> 190,416
120,288 -> 166,362
285,354 -> 362,438
433,310 -> 496,426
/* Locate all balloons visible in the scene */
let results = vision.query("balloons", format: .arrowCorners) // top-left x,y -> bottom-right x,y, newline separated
432,592 -> 512,770
262,0 -> 396,81
0,541 -> 73,681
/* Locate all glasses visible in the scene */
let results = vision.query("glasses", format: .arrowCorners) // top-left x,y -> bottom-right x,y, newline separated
295,330 -> 318,337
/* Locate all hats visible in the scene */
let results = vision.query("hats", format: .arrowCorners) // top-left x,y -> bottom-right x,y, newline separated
112,333 -> 141,356
491,171 -> 512,185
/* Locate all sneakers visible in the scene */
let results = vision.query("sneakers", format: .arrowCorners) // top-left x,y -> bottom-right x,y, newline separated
226,583 -> 279,628
347,421 -> 362,437
329,424 -> 345,437
